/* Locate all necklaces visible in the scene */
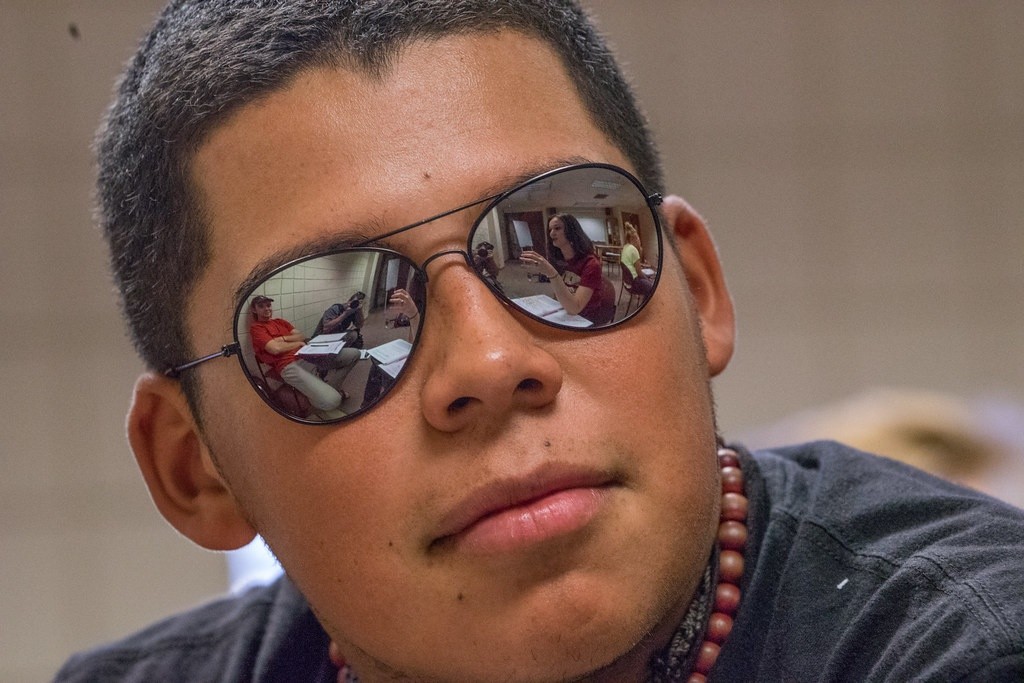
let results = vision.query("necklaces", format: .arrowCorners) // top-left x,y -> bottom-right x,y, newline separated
328,443 -> 750,683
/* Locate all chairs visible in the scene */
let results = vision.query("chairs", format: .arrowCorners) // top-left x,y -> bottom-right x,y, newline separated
256,355 -> 312,415
616,261 -> 647,318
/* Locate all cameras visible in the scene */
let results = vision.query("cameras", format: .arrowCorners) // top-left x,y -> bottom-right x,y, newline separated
478,249 -> 488,258
349,300 -> 359,309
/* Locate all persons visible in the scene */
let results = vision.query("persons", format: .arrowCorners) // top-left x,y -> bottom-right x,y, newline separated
59,0 -> 1024,683
245,204 -> 647,416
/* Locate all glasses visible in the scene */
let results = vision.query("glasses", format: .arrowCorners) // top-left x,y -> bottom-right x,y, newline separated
162,168 -> 662,427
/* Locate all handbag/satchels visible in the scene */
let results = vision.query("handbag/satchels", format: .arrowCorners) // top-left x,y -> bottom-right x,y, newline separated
394,312 -> 410,328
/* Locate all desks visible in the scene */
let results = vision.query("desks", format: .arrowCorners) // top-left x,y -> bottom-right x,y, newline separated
594,245 -> 622,277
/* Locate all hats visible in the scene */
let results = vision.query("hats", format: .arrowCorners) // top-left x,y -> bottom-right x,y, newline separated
251,295 -> 274,308
